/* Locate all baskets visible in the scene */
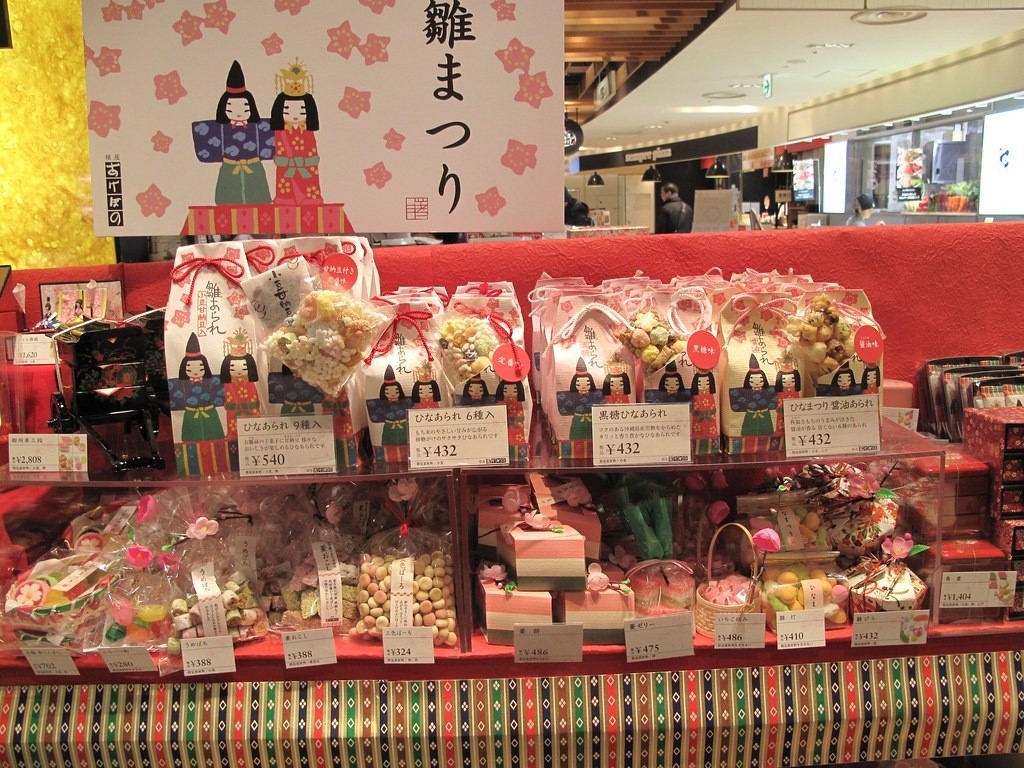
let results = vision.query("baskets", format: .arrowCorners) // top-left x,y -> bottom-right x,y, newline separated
694,522 -> 761,638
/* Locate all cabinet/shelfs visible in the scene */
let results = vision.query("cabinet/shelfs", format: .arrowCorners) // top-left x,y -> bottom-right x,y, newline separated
0,219 -> 1024,768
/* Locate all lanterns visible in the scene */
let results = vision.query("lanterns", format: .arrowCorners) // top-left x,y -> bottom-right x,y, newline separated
564,112 -> 584,156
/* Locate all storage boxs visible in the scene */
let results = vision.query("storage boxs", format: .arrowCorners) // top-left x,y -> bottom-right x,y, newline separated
477,474 -> 637,645
882,351 -> 1024,623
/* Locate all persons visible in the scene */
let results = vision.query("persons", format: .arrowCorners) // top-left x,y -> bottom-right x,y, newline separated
655,183 -> 693,234
847,195 -> 885,226
564,186 -> 595,226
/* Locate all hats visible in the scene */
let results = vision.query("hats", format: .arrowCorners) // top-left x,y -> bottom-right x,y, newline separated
855,194 -> 874,214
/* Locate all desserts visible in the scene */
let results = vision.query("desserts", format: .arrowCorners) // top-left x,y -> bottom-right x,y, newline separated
6,501 -> 363,653
